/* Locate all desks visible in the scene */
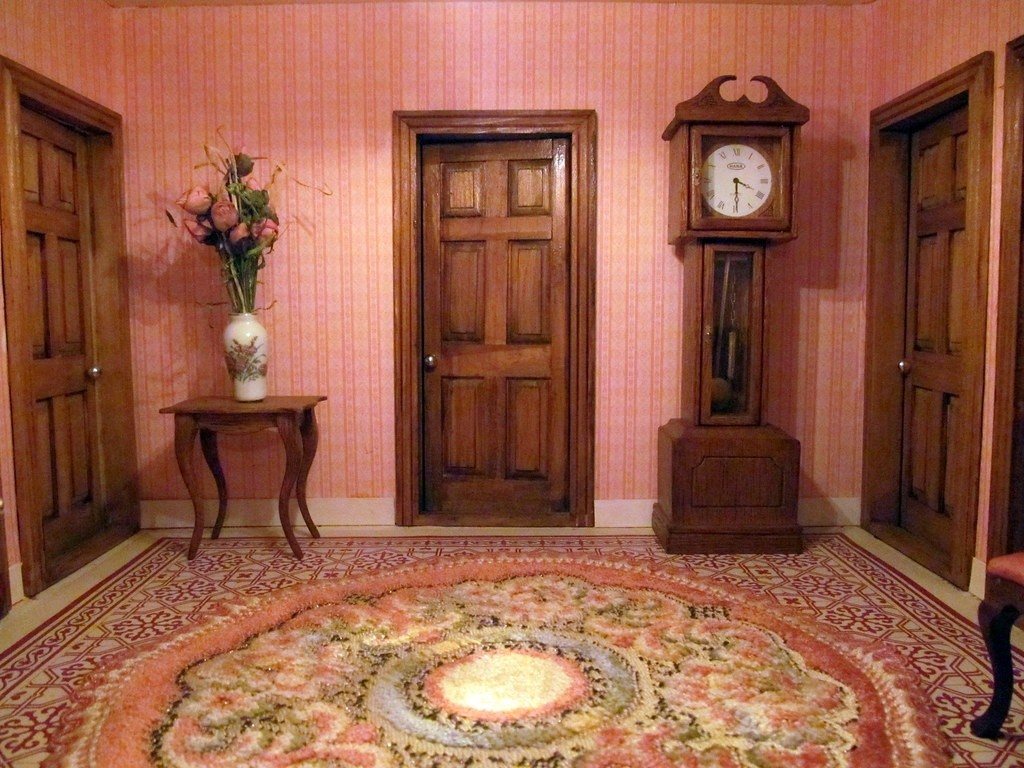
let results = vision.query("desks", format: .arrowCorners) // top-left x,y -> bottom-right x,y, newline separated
158,395 -> 320,559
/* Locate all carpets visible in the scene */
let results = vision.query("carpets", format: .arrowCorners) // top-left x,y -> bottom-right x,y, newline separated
38,552 -> 947,768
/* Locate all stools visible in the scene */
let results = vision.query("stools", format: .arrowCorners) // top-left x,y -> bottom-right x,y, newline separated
969,552 -> 1024,740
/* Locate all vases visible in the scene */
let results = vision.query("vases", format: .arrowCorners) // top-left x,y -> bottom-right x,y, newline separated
222,313 -> 269,402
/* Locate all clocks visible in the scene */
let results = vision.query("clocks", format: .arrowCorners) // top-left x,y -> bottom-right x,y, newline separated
700,140 -> 779,217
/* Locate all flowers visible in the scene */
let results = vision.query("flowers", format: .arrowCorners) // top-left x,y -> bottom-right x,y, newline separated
163,125 -> 333,313
